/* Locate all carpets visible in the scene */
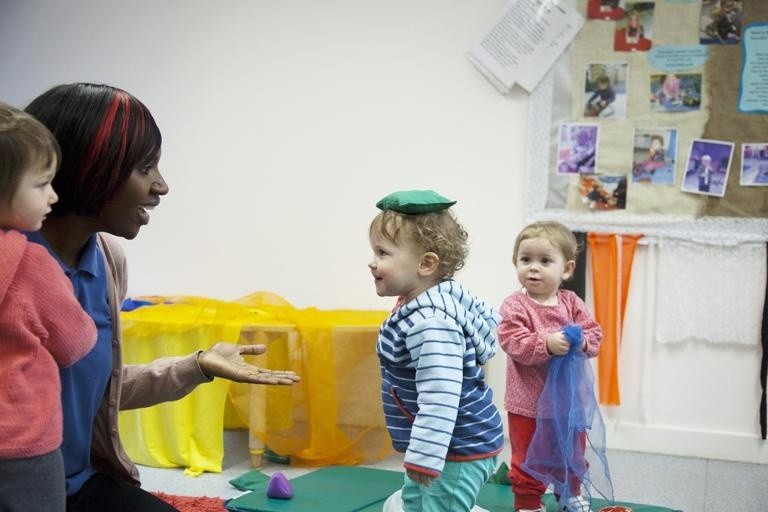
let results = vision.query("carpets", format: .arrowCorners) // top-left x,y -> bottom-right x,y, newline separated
151,492 -> 229,511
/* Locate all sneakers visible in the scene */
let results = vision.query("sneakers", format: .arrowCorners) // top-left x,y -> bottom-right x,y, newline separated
558,495 -> 592,512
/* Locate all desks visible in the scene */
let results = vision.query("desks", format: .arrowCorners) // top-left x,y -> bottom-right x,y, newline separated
119,304 -> 392,471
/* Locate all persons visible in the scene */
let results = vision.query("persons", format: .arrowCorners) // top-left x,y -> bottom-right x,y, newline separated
619,9 -> 646,47
743,146 -> 754,158
647,135 -> 667,162
660,72 -> 682,101
0,98 -> 100,511
697,154 -> 715,192
759,145 -> 768,160
17,78 -> 305,511
563,127 -> 596,174
588,75 -> 617,113
582,181 -> 609,209
490,217 -> 606,512
365,207 -> 509,511
713,0 -> 741,41
